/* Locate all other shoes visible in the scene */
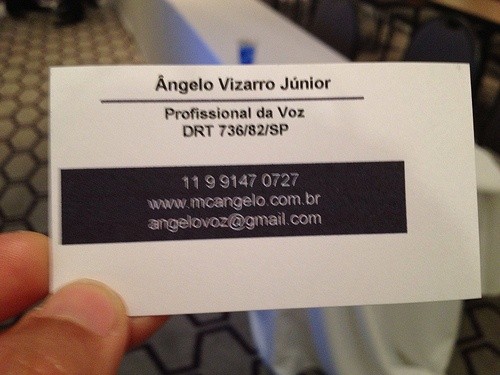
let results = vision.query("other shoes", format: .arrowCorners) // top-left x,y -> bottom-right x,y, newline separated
5,1 -> 100,27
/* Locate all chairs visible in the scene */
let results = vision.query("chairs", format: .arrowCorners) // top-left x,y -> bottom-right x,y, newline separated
306,0 -> 357,60
399,18 -> 479,102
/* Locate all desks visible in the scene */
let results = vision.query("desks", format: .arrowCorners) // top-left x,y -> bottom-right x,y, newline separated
113,1 -> 500,375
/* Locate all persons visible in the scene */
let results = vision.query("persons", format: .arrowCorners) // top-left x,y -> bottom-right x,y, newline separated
1,0 -> 92,28
0,229 -> 171,375
